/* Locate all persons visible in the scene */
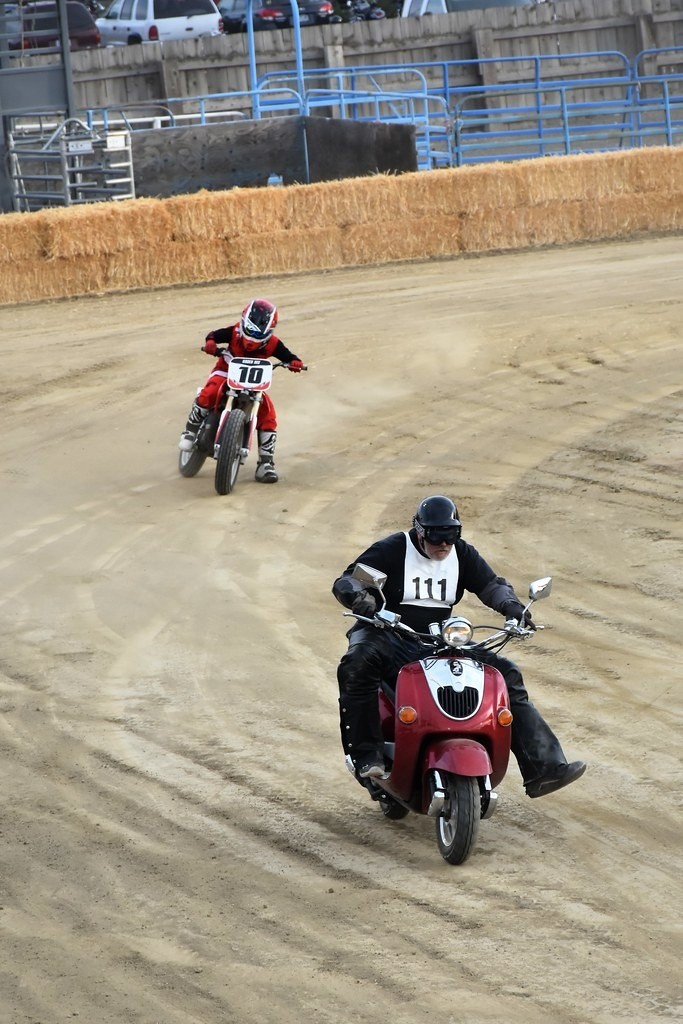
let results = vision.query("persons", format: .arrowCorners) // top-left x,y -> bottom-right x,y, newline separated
178,298 -> 303,483
332,495 -> 587,798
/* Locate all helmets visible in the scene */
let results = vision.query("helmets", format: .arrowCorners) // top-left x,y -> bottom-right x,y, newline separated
418,496 -> 461,526
240,300 -> 278,343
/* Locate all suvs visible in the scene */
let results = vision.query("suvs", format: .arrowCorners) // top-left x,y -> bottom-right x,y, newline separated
215,0 -> 334,31
3,0 -> 102,55
97,0 -> 225,47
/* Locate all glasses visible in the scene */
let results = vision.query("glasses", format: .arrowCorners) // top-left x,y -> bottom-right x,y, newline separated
415,519 -> 459,546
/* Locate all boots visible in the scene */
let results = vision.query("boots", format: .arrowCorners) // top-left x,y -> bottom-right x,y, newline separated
255,430 -> 278,483
178,397 -> 209,452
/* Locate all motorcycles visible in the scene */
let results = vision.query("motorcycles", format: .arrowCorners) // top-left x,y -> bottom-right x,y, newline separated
340,562 -> 552,865
179,346 -> 308,497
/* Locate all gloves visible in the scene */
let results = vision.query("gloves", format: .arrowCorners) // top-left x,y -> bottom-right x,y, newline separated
503,603 -> 537,631
352,590 -> 376,622
206,340 -> 216,354
291,360 -> 303,373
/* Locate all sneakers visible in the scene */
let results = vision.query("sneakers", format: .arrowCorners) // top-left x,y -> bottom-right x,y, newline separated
360,762 -> 385,777
526,761 -> 586,798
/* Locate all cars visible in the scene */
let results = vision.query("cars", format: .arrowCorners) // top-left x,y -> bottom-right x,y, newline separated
403,0 -> 546,18
